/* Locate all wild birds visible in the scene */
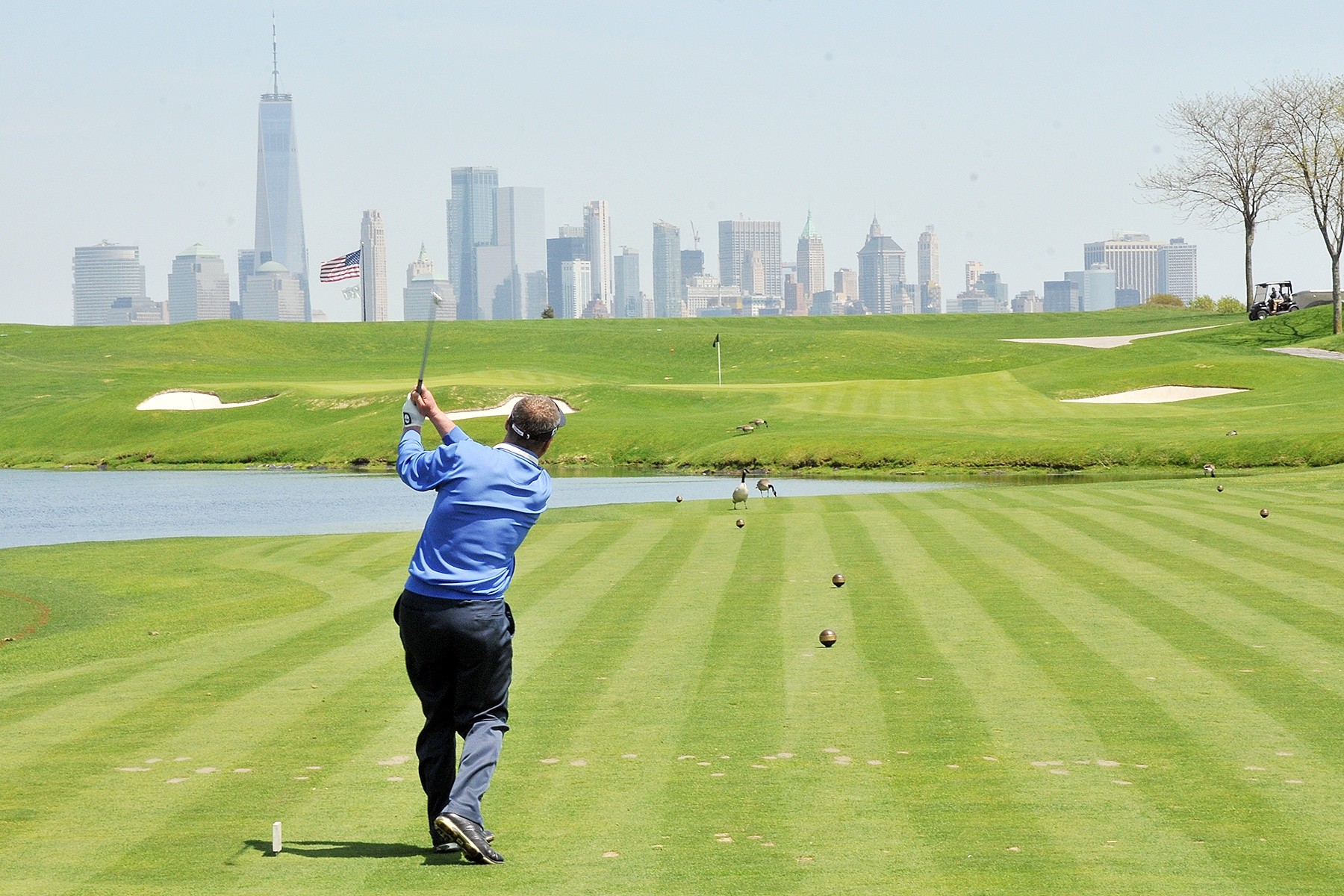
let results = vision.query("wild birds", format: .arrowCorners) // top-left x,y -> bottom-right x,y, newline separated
755,479 -> 777,498
736,418 -> 768,435
1201,463 -> 1215,478
1226,430 -> 1237,436
732,469 -> 750,510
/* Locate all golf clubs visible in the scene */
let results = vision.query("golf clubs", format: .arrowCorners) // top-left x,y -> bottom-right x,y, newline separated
414,291 -> 442,405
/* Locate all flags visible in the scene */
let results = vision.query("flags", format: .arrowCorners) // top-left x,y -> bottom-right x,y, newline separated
320,249 -> 361,282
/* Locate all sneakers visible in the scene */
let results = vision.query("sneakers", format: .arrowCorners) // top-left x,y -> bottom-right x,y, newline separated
434,812 -> 505,865
428,830 -> 495,853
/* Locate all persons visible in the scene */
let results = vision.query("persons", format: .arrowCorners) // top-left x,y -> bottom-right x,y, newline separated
1269,287 -> 1284,313
396,383 -> 566,864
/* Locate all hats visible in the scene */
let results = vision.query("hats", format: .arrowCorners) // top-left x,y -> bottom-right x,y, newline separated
507,395 -> 566,441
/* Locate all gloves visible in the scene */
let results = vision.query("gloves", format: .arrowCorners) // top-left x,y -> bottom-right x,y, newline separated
402,392 -> 425,428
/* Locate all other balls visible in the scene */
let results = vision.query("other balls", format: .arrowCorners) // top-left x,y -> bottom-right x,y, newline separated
677,496 -> 682,502
736,519 -> 744,528
819,630 -> 837,647
1260,509 -> 1269,518
832,574 -> 845,587
1217,485 -> 1223,492
